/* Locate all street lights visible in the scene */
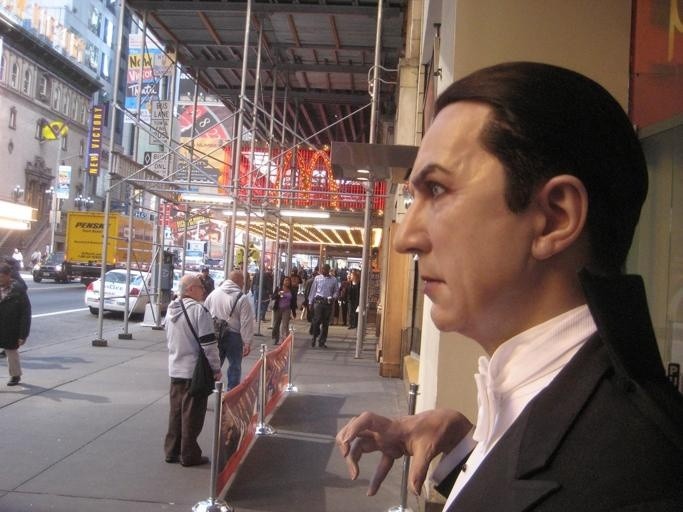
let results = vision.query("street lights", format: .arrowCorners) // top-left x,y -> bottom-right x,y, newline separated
85,197 -> 95,211
12,185 -> 23,204
194,219 -> 205,241
44,186 -> 54,212
214,226 -> 224,256
49,151 -> 100,252
74,194 -> 85,210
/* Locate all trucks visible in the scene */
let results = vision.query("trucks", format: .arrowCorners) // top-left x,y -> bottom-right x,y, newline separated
65,210 -> 181,287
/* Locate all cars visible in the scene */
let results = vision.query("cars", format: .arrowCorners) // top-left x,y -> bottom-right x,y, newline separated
83,270 -> 156,316
168,251 -> 298,298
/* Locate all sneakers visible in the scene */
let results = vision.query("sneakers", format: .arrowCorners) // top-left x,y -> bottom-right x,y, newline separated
7,375 -> 21,386
272,337 -> 295,345
166,454 -> 209,467
310,335 -> 328,350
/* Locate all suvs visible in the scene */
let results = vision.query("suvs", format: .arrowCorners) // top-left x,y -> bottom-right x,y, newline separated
34,250 -> 69,284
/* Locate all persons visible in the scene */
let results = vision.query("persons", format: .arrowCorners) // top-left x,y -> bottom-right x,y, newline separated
0,260 -> 30,386
198,263 -> 361,349
163,272 -> 223,467
0,254 -> 20,358
201,270 -> 253,391
336,58 -> 682,511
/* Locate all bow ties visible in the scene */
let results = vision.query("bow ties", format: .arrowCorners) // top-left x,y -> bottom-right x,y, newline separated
471,354 -> 502,457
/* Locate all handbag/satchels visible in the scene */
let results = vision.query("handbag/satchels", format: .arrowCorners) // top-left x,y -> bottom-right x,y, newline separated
212,315 -> 227,339
186,351 -> 216,396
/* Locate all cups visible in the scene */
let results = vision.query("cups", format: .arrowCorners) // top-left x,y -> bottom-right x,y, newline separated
279,291 -> 284,298
328,297 -> 332,304
